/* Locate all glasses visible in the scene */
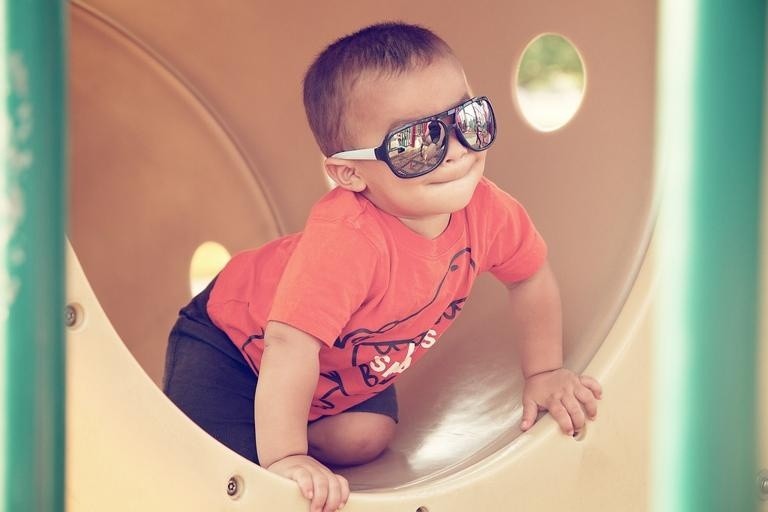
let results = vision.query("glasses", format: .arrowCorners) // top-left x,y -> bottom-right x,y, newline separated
332,95 -> 495,179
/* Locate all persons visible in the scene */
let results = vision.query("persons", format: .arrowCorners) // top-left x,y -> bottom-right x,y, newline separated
161,20 -> 603,512
422,121 -> 445,165
